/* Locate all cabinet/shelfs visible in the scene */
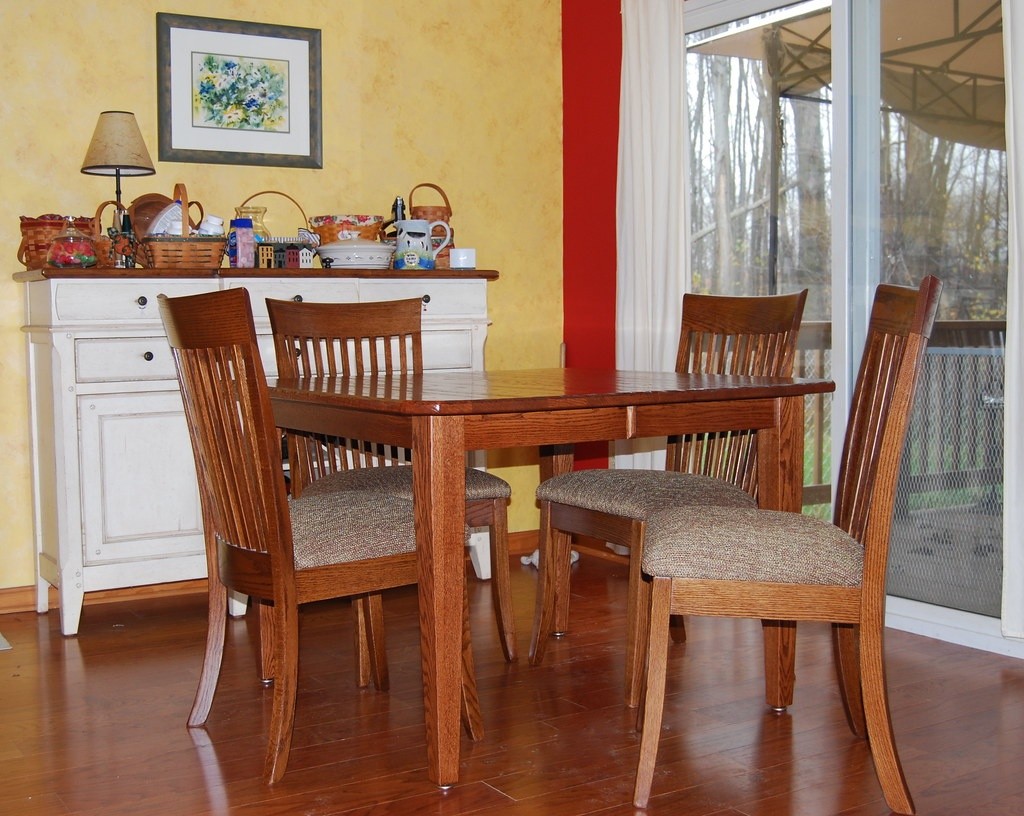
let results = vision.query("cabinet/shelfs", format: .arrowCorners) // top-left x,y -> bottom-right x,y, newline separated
13,266 -> 499,637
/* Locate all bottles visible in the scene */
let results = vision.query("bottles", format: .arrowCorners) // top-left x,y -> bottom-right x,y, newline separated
121,210 -> 135,269
112,209 -> 122,268
235,206 -> 271,242
45,217 -> 98,268
197,214 -> 225,237
146,200 -> 182,234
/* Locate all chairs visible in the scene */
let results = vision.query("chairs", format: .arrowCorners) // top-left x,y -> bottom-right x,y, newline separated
155,286 -> 485,785
634,274 -> 943,815
527,289 -> 810,707
263,295 -> 513,688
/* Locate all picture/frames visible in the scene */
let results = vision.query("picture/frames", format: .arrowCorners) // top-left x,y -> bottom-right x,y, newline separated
155,10 -> 323,171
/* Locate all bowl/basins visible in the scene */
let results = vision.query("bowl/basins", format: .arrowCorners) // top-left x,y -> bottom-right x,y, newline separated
315,231 -> 397,269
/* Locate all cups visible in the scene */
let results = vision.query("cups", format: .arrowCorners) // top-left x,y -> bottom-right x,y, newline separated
393,219 -> 451,270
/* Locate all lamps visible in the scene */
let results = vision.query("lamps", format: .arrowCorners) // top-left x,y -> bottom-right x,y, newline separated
81,110 -> 156,214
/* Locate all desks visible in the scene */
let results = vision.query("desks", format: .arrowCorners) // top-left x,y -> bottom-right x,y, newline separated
234,369 -> 837,790
